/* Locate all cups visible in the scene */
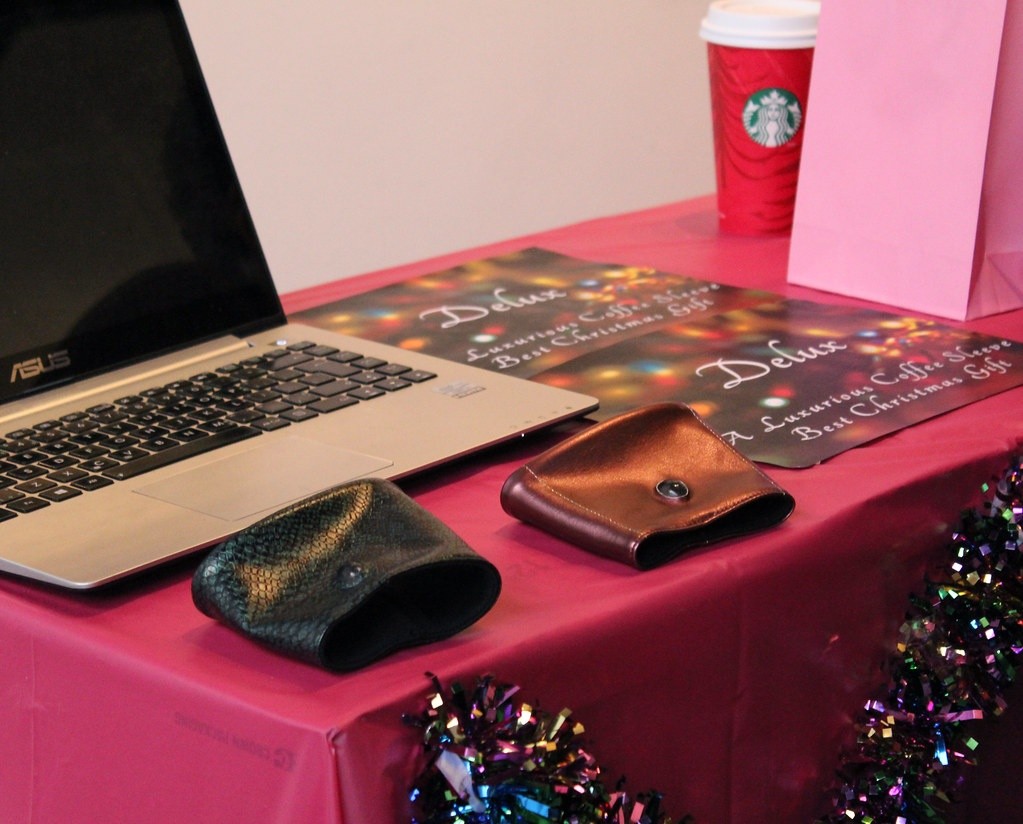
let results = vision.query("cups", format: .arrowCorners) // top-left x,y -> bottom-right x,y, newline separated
701,1 -> 822,236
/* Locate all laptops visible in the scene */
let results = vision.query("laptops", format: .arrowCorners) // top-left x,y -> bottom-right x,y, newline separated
0,1 -> 601,592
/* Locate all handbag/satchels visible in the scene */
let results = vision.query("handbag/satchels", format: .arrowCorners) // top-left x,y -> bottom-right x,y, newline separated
782,0 -> 1023,324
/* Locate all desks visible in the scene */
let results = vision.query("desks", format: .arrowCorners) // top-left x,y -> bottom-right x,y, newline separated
1,187 -> 1023,823
